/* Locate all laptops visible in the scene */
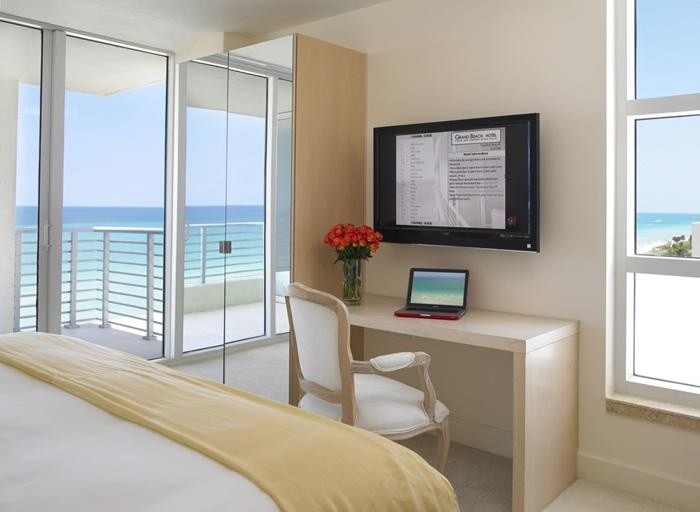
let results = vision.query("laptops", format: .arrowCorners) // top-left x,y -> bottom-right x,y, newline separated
394,267 -> 469,319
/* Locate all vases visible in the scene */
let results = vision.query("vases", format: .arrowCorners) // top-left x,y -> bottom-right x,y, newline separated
341,258 -> 364,307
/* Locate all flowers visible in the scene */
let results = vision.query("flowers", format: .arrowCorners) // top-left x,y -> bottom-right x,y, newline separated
324,223 -> 384,298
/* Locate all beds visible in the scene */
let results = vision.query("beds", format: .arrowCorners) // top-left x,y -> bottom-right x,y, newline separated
1,329 -> 461,512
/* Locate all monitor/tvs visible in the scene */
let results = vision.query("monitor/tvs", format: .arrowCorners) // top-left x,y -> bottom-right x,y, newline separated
371,112 -> 541,252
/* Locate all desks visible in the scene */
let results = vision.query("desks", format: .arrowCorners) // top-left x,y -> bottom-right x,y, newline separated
346,291 -> 580,512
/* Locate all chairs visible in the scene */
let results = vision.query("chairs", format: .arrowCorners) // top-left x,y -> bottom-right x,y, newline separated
282,281 -> 451,474
